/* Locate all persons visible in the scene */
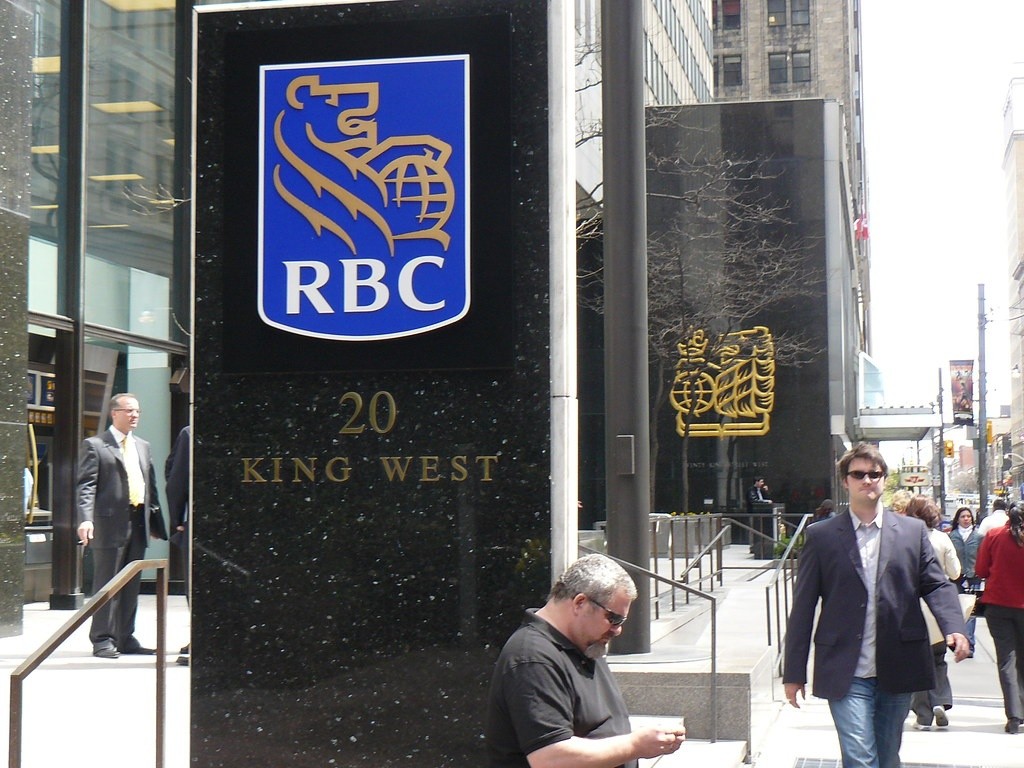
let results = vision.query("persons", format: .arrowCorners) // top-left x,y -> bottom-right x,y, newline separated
482,553 -> 688,768
76,393 -> 168,660
748,475 -> 773,554
782,443 -> 971,768
164,423 -> 189,666
889,488 -> 1024,734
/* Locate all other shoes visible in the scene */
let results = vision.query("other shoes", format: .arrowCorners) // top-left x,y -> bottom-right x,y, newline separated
933,705 -> 949,726
967,645 -> 974,658
913,721 -> 931,731
180,643 -> 190,654
117,646 -> 158,654
175,656 -> 189,664
94,648 -> 120,657
1005,718 -> 1020,734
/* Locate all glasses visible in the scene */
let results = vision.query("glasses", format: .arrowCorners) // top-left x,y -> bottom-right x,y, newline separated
113,408 -> 141,416
572,592 -> 631,626
846,470 -> 888,479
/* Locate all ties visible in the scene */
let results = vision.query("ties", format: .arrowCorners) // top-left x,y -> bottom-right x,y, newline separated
121,436 -> 138,507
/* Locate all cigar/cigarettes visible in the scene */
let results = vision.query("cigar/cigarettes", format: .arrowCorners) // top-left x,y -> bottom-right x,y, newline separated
78,538 -> 88,543
